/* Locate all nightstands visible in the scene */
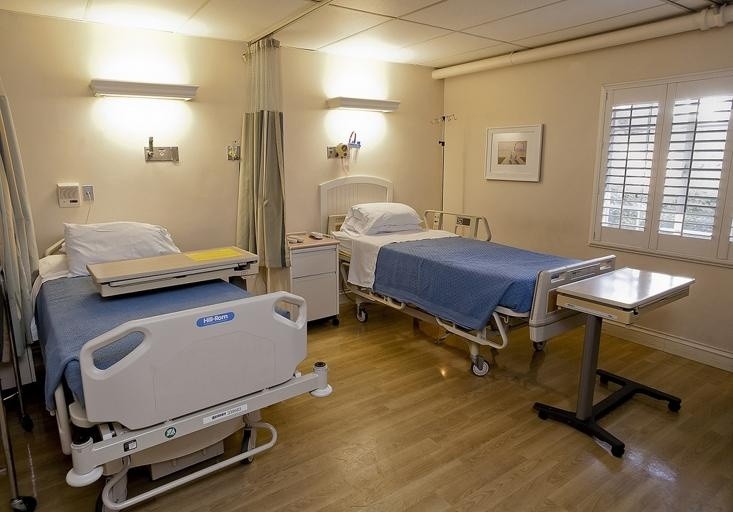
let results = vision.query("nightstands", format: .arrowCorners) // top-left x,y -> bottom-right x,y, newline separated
275,229 -> 338,327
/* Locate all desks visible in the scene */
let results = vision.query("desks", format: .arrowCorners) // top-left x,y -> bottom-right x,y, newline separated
531,264 -> 695,455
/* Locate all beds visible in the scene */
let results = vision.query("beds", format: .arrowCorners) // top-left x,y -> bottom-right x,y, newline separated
30,221 -> 334,503
321,175 -> 618,379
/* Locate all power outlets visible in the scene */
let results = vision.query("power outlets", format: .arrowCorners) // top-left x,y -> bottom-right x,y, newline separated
327,144 -> 338,163
83,186 -> 96,207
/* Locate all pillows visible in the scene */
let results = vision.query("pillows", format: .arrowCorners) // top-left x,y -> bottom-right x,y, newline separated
60,221 -> 182,279
345,203 -> 421,225
347,221 -> 426,236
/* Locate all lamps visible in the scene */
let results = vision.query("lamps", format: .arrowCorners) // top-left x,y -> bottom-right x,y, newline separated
338,129 -> 362,165
327,96 -> 401,113
87,78 -> 199,103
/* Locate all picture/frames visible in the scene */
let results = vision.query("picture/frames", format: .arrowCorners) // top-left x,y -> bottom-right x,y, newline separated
484,123 -> 544,184
